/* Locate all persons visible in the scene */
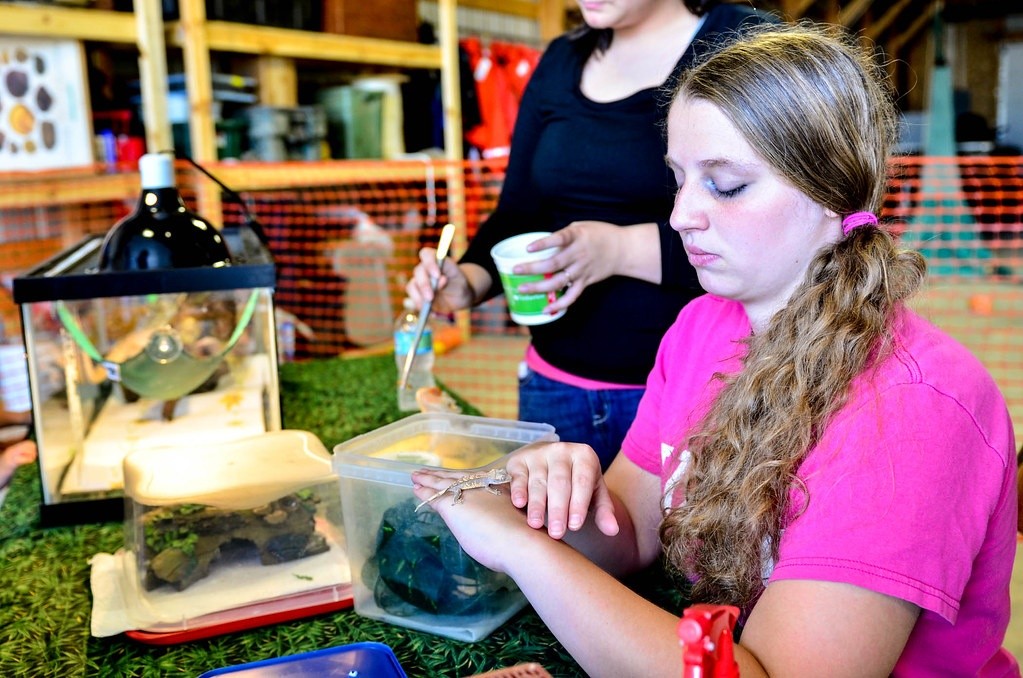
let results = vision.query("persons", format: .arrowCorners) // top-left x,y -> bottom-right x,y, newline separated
409,21 -> 1022,678
401,0 -> 786,487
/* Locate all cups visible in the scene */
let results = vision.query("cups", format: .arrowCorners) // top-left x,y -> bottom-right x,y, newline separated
490,231 -> 566,325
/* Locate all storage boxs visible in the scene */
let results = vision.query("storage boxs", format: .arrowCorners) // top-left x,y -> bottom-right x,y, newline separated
12,224 -> 279,526
330,411 -> 560,628
123,429 -> 353,645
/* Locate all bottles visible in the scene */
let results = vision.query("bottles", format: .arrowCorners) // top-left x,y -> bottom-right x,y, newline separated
394,297 -> 437,413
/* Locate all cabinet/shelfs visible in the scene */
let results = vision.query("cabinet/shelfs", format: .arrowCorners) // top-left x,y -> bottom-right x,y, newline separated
0,0 -> 175,213
166,0 -> 474,354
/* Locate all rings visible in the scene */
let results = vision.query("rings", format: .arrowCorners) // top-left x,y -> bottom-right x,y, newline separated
566,272 -> 573,287
